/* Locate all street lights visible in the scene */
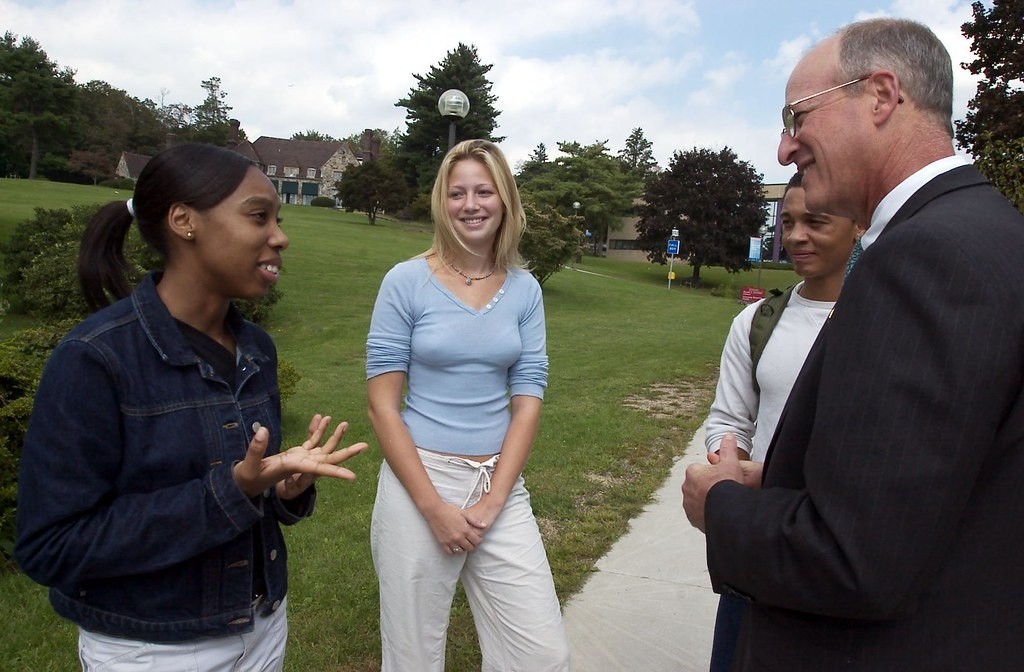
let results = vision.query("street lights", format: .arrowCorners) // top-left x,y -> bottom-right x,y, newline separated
572,200 -> 582,215
756,226 -> 768,289
437,89 -> 470,154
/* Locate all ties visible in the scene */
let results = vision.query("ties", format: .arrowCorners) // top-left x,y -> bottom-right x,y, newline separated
844,238 -> 863,281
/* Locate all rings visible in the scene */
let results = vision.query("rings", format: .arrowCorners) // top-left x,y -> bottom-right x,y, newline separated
453,547 -> 460,553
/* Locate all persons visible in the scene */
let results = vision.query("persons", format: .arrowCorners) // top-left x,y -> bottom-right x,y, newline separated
365,138 -> 570,672
680,17 -> 1022,672
703,173 -> 866,465
12,143 -> 368,672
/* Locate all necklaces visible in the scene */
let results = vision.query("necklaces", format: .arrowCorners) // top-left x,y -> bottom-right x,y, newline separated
450,261 -> 497,286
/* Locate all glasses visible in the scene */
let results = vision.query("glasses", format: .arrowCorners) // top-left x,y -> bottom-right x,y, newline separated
781,68 -> 905,142
